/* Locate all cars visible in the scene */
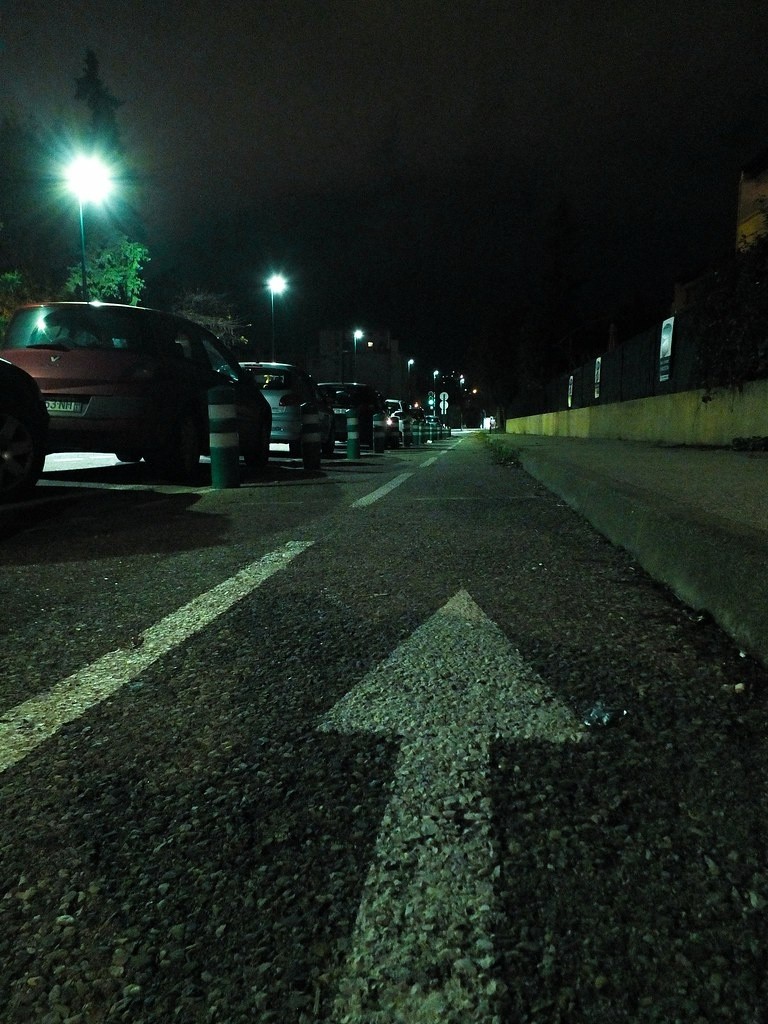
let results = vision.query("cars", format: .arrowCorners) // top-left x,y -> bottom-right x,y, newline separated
425,414 -> 439,427
232,357 -> 341,464
0,357 -> 51,498
319,381 -> 386,452
6,297 -> 274,479
380,397 -> 409,426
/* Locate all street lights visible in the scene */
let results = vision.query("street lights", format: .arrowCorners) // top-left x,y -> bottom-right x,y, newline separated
265,271 -> 290,322
54,143 -> 124,303
353,329 -> 364,365
